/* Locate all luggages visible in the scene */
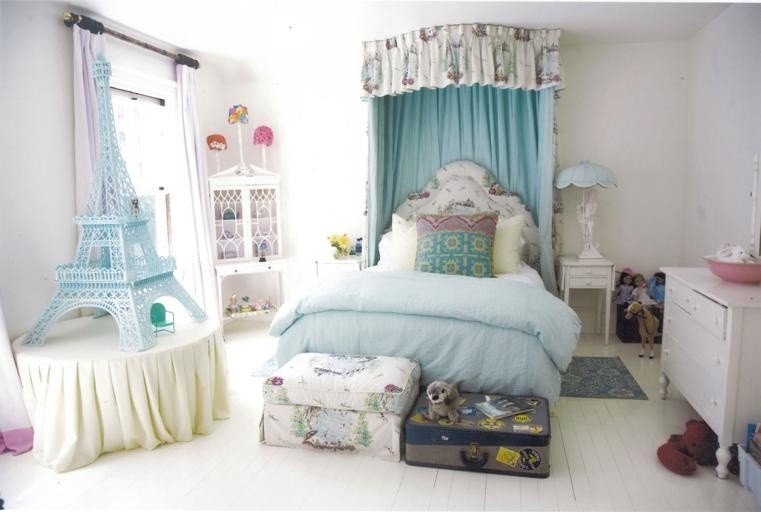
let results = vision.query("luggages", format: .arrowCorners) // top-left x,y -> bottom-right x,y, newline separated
402,387 -> 553,479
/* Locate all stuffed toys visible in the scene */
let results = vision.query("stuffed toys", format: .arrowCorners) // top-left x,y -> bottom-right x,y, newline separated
654,419 -> 741,478
424,379 -> 462,423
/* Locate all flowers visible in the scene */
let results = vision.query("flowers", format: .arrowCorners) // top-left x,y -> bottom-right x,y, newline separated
327,230 -> 349,258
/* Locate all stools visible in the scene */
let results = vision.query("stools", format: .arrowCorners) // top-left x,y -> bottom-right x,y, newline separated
262,351 -> 423,464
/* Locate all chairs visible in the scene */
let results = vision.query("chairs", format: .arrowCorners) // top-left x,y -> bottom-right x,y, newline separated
149,301 -> 177,338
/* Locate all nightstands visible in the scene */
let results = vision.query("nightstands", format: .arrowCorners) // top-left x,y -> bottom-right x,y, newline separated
555,254 -> 617,347
310,257 -> 361,278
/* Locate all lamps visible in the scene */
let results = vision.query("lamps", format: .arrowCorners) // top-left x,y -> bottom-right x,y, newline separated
553,159 -> 620,258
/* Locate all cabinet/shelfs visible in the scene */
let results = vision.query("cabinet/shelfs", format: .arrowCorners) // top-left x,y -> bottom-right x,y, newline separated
657,266 -> 761,480
207,160 -> 285,337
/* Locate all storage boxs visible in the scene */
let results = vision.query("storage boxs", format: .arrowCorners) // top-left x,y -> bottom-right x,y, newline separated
736,442 -> 761,509
614,302 -> 663,345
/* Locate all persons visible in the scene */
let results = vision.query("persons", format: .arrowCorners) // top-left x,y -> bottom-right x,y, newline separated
612,266 -> 666,357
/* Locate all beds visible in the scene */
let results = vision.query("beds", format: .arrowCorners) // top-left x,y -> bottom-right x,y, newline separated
269,20 -> 582,404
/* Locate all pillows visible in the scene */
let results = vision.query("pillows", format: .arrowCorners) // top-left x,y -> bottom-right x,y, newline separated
413,212 -> 498,278
389,213 -> 527,276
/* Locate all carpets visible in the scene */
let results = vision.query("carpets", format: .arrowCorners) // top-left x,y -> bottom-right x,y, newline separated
561,355 -> 648,400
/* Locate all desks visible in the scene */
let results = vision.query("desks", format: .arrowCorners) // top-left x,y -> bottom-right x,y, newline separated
8,309 -> 232,472
